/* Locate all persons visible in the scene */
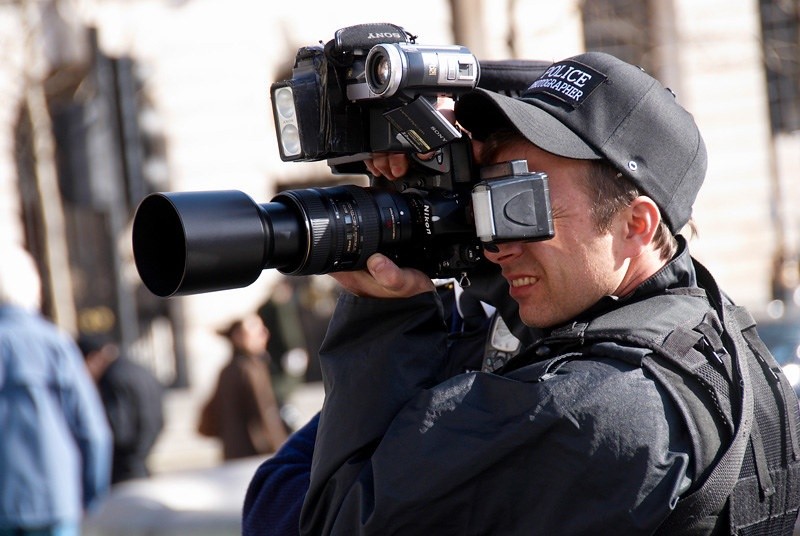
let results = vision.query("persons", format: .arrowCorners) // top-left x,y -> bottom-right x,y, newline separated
300,51 -> 800,536
0,244 -> 335,536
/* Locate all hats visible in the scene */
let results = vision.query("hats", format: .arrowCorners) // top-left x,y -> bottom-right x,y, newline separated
453,52 -> 708,236
